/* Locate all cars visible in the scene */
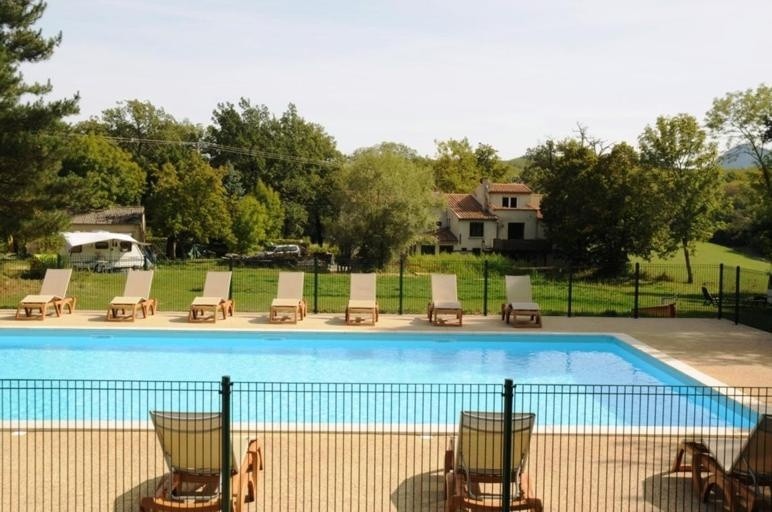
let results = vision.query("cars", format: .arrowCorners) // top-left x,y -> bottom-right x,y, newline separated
264,244 -> 301,258
766,274 -> 772,304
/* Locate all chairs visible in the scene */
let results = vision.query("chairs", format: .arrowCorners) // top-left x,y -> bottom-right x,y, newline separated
17,267 -> 77,322
188,271 -> 235,324
428,272 -> 463,327
270,271 -> 307,325
140,412 -> 263,511
441,412 -> 544,511
671,413 -> 771,510
106,268 -> 158,322
344,272 -> 379,326
501,275 -> 542,328
